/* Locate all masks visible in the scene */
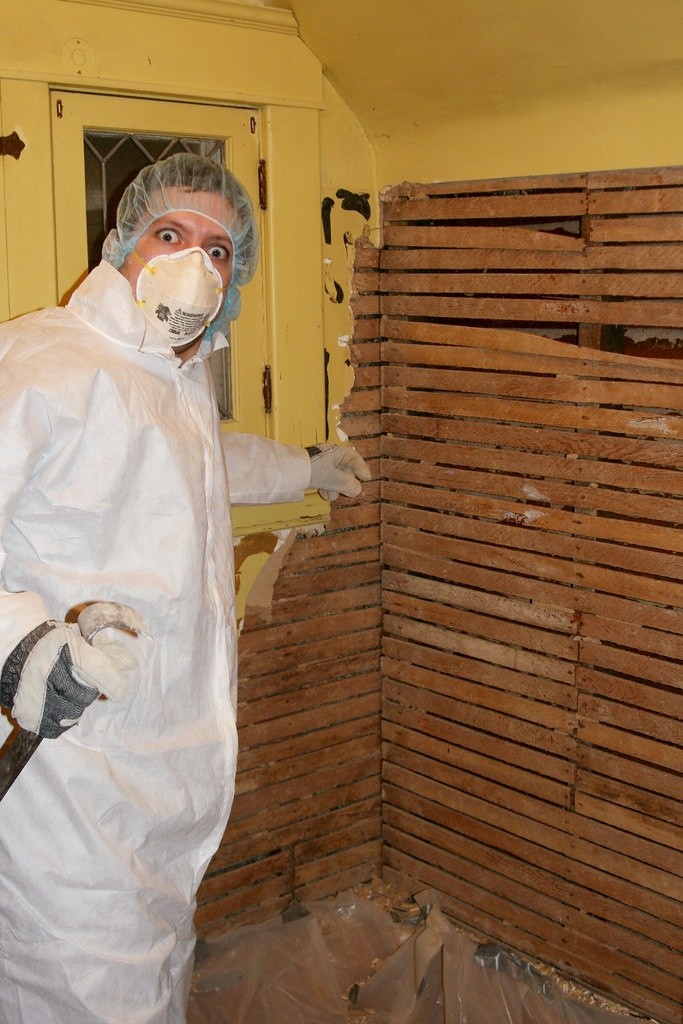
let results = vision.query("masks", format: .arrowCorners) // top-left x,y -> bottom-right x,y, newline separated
123,246 -> 227,348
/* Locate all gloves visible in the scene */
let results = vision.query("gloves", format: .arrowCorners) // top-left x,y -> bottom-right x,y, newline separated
305,443 -> 372,502
0,620 -> 129,739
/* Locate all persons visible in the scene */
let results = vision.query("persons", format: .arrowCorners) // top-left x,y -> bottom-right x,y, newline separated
0,151 -> 371,1024
